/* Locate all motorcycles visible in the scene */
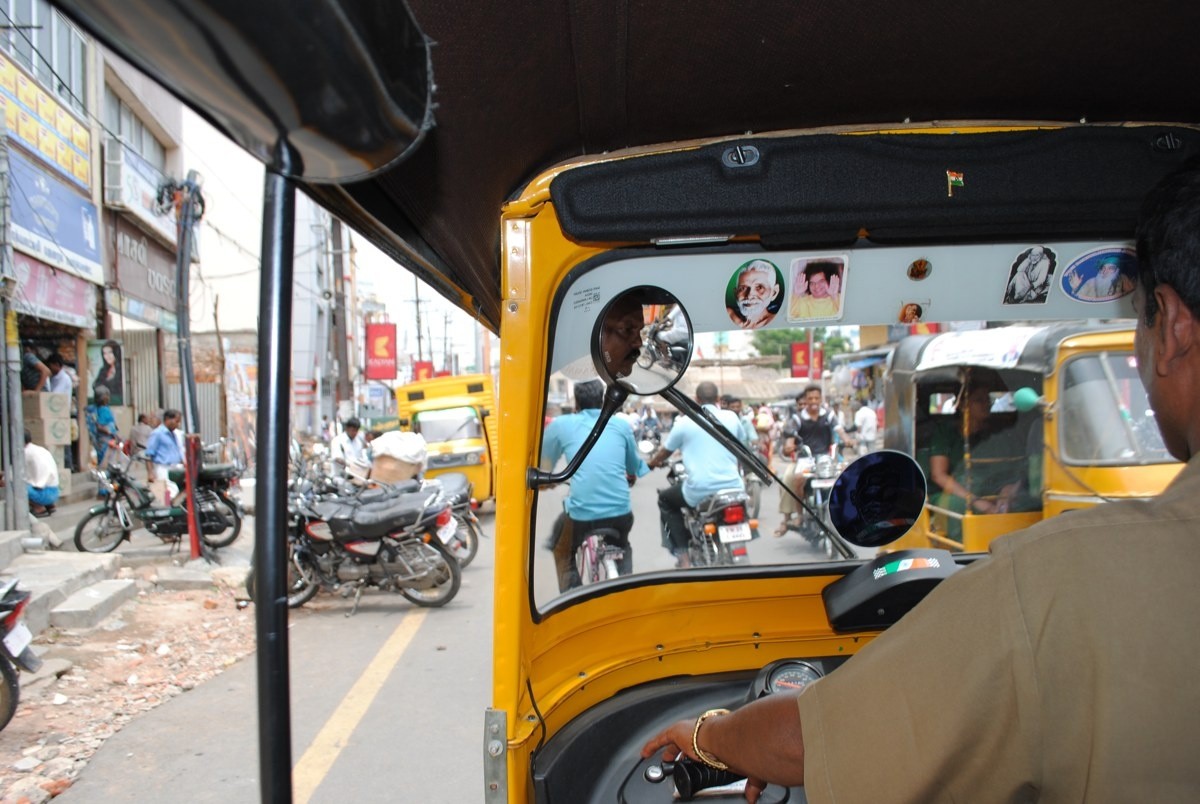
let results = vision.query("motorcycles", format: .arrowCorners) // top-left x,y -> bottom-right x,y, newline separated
396,371 -> 498,517
246,439 -> 483,620
863,321 -> 1187,568
636,439 -> 760,567
782,438 -> 855,559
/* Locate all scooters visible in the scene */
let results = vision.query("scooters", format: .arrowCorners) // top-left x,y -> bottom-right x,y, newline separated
0,574 -> 46,733
75,428 -> 250,556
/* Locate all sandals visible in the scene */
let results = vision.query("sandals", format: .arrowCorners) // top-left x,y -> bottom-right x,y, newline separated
774,522 -> 789,537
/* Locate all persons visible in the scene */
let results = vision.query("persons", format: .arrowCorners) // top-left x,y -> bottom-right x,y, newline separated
790,262 -> 841,318
539,380 -> 651,595
129,407 -> 187,542
1008,246 -> 1050,302
599,292 -> 645,378
615,381 -> 882,563
915,378 -> 1128,545
93,341 -> 123,405
330,418 -> 381,484
10,427 -> 60,517
87,385 -> 121,500
21,339 -> 79,472
1068,256 -> 1133,299
639,159 -> 1200,804
726,259 -> 780,329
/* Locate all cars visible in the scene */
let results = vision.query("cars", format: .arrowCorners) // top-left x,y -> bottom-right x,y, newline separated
635,303 -> 691,374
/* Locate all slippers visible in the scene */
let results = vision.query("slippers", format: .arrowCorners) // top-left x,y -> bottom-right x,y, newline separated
47,506 -> 56,513
31,509 -> 49,517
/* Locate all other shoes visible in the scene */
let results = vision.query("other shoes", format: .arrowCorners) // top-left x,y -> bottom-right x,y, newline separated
788,514 -> 803,530
659,361 -> 672,369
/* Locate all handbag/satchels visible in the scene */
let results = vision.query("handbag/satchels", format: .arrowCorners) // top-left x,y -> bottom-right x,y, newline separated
145,480 -> 170,506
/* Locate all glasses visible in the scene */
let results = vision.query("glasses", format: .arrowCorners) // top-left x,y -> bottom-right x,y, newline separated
970,396 -> 995,406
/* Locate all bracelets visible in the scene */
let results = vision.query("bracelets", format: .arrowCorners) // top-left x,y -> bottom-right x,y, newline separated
692,707 -> 732,772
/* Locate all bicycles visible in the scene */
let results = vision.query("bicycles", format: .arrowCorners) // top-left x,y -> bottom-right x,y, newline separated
540,482 -> 624,588
